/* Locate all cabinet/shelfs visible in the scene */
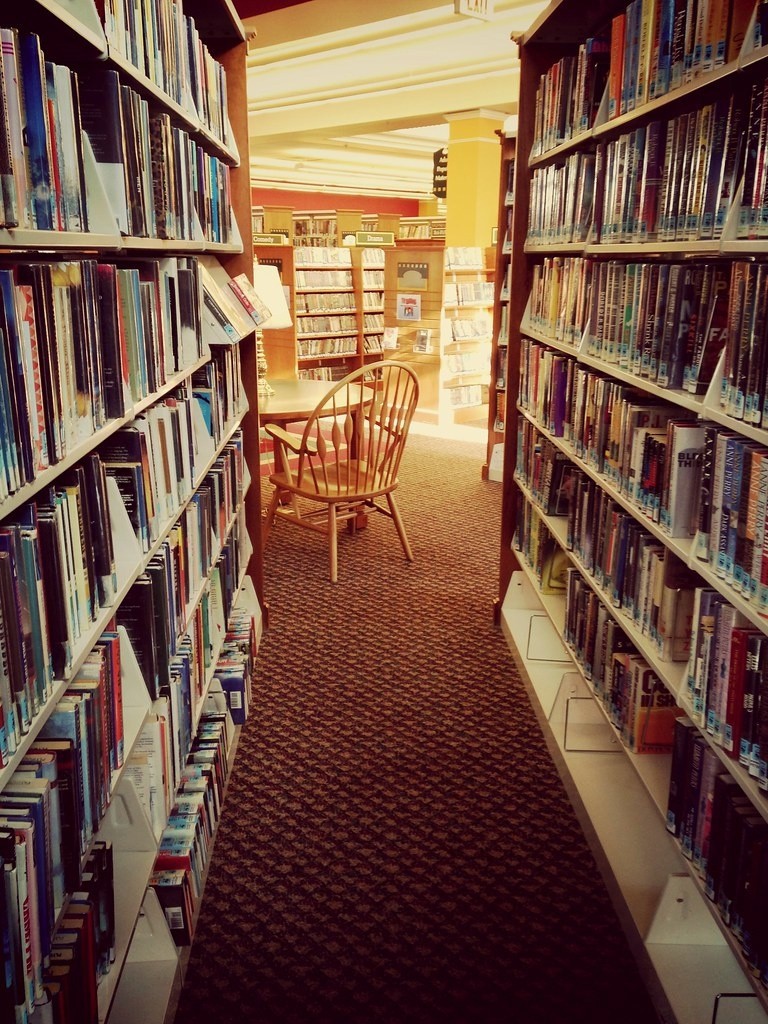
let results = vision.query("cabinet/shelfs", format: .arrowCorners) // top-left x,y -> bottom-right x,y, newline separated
0,0 -> 275,1024
481,127 -> 518,484
252,206 -> 495,426
490,0 -> 768,1024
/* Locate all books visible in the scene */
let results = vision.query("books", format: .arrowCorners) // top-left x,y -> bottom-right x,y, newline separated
299,364 -> 349,381
361,222 -> 378,232
444,246 -> 494,409
361,248 -> 384,381
399,223 -> 446,239
98,0 -> 229,146
510,487 -> 768,1003
525,81 -> 768,243
530,0 -> 768,159
513,414 -> 768,801
0,254 -> 272,502
488,159 -> 515,483
519,255 -> 768,431
292,220 -> 337,247
0,342 -> 248,768
511,336 -> 768,623
0,25 -> 232,245
294,248 -> 359,359
1,424 -> 257,1024
251,215 -> 264,233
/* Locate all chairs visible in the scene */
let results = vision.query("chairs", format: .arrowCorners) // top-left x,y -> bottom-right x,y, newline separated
265,358 -> 421,583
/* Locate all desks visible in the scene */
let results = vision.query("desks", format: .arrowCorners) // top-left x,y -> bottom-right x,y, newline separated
257,370 -> 377,532
